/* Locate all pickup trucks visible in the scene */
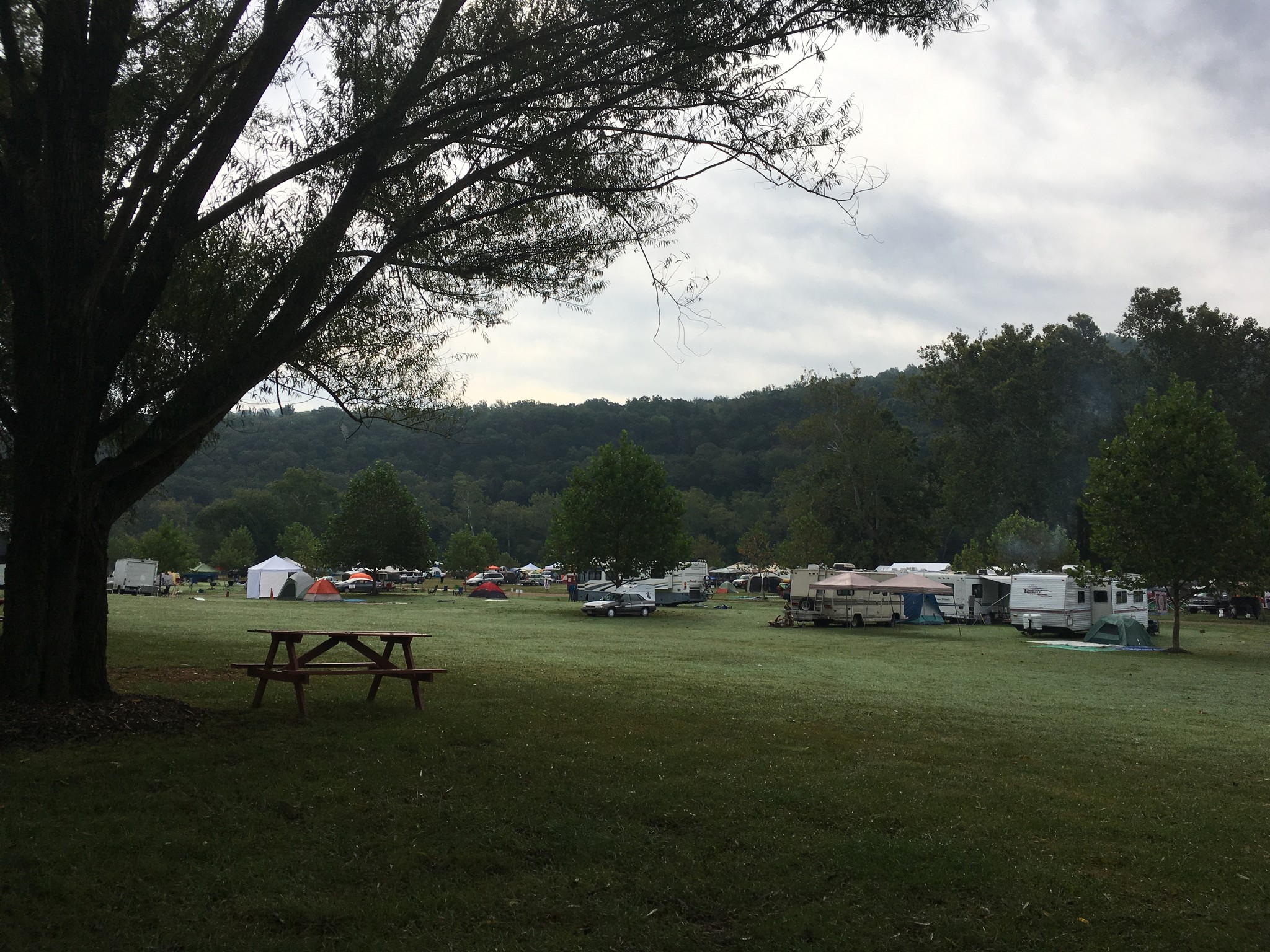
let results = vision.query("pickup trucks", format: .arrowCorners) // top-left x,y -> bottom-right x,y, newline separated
418,570 -> 436,579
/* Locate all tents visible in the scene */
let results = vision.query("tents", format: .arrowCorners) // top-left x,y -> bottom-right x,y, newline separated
461,562 -> 576,600
1081,587 -> 1168,647
707,562 -> 791,597
155,555 -> 424,604
803,563 -> 961,637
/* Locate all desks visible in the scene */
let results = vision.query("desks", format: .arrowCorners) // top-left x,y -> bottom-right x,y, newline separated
158,587 -> 165,592
172,582 -> 192,591
247,628 -> 433,716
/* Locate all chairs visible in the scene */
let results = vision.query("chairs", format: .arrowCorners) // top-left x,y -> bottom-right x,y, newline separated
223,581 -> 234,590
453,585 -> 459,592
385,583 -> 392,592
454,587 -> 463,597
411,583 -> 417,592
441,586 -> 448,594
428,585 -> 438,596
402,585 -> 409,594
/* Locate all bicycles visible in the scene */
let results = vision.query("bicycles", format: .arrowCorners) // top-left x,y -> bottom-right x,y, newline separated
702,575 -> 714,599
568,585 -> 577,602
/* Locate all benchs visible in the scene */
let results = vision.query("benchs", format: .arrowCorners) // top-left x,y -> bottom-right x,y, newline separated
106,583 -> 115,594
137,585 -> 159,596
283,667 -> 448,676
230,661 -> 377,669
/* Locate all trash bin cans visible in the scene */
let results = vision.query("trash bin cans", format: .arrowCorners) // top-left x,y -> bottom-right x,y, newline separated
568,584 -> 578,600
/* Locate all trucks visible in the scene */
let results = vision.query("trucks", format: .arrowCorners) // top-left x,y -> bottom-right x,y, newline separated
782,562 -> 909,628
108,559 -> 159,596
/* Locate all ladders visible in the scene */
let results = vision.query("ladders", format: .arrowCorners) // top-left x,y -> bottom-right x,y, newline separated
814,564 -> 827,612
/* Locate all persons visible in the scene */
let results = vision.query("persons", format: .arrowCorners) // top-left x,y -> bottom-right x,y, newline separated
440,571 -> 445,583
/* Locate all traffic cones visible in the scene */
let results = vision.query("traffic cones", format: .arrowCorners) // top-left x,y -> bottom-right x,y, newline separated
269,588 -> 274,600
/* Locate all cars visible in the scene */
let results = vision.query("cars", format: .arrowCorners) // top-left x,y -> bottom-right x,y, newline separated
581,592 -> 656,618
1228,596 -> 1264,620
312,576 -> 342,584
228,566 -> 251,578
333,578 -> 383,594
181,573 -> 218,583
516,570 -> 554,586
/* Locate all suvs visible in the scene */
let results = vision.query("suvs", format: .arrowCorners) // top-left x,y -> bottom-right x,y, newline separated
540,572 -> 560,582
393,572 -> 425,584
467,573 -> 504,587
731,574 -> 753,592
